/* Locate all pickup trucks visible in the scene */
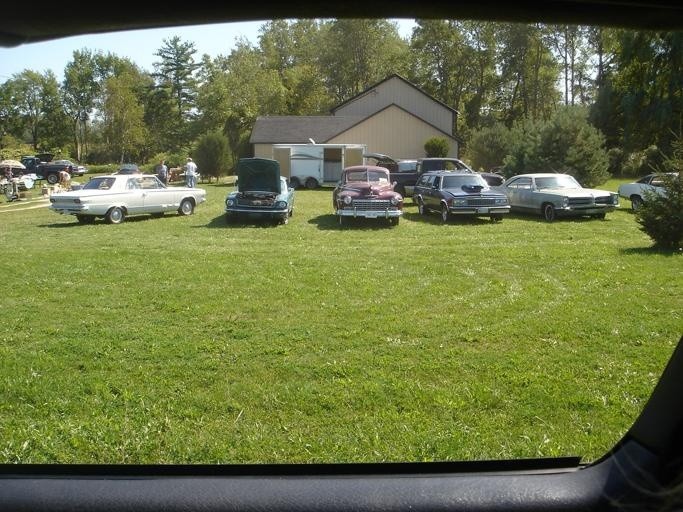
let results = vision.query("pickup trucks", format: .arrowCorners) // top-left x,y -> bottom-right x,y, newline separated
382,156 -> 506,198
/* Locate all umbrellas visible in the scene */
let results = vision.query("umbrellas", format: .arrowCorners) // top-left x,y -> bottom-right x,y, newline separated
0,159 -> 27,178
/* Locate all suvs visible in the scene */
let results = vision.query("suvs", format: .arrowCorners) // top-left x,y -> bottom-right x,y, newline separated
361,150 -> 420,172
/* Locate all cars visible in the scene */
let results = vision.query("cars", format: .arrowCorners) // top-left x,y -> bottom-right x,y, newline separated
224,157 -> 298,225
0,153 -> 88,185
49,165 -> 208,223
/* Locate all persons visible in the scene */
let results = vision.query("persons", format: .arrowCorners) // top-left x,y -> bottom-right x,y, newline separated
3,166 -> 14,182
56,170 -> 70,188
156,159 -> 167,187
184,158 -> 197,188
